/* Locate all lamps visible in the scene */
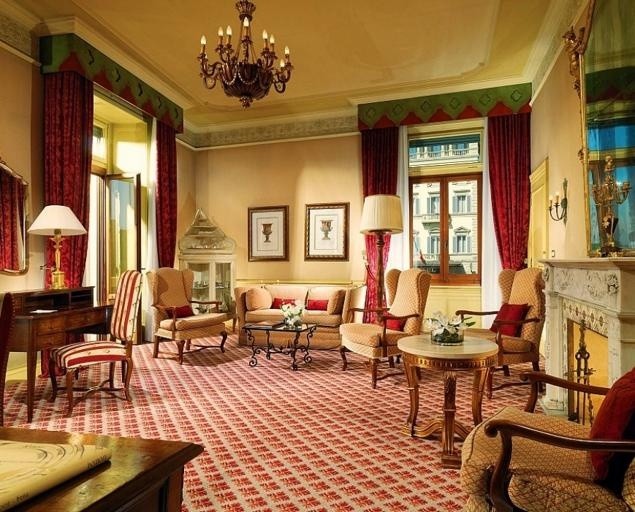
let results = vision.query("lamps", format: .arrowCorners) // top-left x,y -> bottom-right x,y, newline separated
27,204 -> 89,288
548,177 -> 568,227
197,1 -> 293,111
360,194 -> 405,323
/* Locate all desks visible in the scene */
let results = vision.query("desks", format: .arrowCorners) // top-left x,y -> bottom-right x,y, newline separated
397,334 -> 499,467
0,286 -> 127,424
0,426 -> 203,512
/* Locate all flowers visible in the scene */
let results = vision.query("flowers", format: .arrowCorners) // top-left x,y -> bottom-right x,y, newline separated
424,311 -> 475,334
281,302 -> 304,326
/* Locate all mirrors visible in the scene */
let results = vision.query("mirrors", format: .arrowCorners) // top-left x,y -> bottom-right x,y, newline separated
1,158 -> 29,276
561,0 -> 635,258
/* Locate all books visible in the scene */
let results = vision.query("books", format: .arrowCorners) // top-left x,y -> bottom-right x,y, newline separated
251,320 -> 285,328
0,438 -> 113,511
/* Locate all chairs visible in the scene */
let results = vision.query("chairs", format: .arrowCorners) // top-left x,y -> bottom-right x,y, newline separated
461,370 -> 635,511
338,268 -> 431,391
144,267 -> 228,365
48,269 -> 143,417
456,267 -> 545,397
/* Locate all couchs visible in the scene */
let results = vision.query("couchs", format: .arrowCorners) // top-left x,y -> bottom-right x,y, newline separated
235,286 -> 367,350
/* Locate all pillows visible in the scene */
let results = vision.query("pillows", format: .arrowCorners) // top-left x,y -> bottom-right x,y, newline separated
490,301 -> 528,336
377,310 -> 406,330
165,305 -> 195,320
269,296 -> 298,310
306,299 -> 329,310
589,367 -> 635,476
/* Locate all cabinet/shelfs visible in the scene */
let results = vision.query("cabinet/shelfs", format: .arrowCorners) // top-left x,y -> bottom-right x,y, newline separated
177,252 -> 236,327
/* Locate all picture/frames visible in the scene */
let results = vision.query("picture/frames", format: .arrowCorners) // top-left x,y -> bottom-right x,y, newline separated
304,204 -> 348,261
247,205 -> 289,262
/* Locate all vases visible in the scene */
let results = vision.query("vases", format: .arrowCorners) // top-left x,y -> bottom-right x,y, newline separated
430,330 -> 465,346
283,315 -> 303,327
